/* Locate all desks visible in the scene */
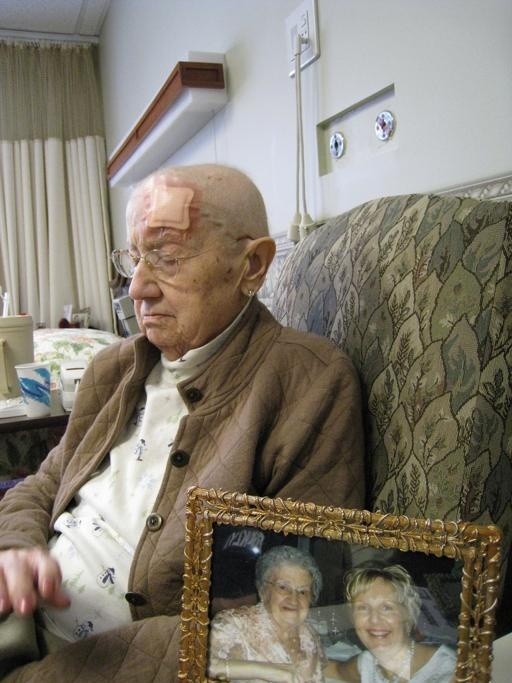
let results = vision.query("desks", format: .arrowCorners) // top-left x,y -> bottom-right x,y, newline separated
0,390 -> 71,434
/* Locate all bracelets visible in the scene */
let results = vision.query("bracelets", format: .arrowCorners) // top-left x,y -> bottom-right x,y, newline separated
223,657 -> 235,683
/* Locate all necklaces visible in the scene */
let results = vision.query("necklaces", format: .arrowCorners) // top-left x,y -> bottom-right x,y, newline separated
373,638 -> 416,683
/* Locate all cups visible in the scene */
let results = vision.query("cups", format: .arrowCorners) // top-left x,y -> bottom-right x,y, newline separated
0,313 -> 34,404
13,360 -> 53,419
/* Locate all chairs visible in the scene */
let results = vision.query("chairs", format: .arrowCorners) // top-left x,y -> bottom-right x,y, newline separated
0,189 -> 511,680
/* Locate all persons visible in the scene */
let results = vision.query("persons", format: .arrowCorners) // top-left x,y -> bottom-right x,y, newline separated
322,559 -> 460,683
1,157 -> 367,683
210,543 -> 329,683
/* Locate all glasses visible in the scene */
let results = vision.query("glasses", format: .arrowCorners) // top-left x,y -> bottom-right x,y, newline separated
262,575 -> 316,600
111,235 -> 257,278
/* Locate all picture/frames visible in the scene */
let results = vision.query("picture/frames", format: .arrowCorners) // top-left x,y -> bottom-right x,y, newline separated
172,482 -> 504,682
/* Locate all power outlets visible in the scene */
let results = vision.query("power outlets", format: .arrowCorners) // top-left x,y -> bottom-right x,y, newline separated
285,0 -> 321,78
